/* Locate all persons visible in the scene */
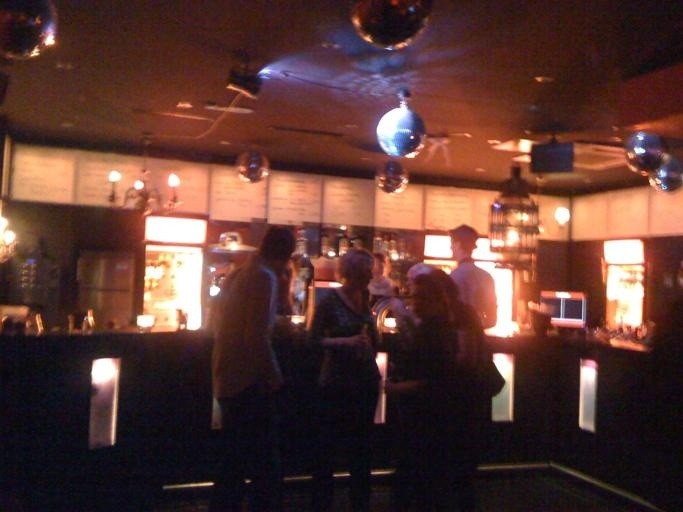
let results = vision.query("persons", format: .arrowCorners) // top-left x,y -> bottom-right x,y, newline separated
212,225 -> 296,511
448,225 -> 498,330
384,264 -> 504,510
307,249 -> 381,511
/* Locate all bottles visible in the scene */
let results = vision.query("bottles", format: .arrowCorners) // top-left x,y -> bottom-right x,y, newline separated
320,230 -> 361,257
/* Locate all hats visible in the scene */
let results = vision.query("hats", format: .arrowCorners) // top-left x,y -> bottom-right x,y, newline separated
449,224 -> 480,248
